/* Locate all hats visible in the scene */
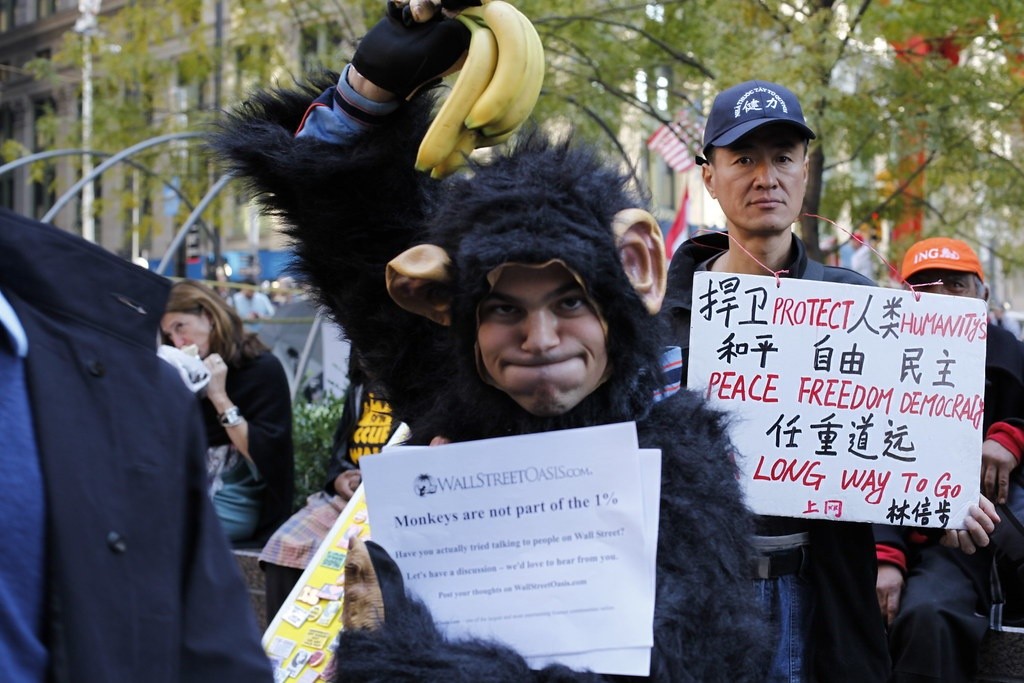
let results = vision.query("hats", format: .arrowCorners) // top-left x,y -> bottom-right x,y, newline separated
899,238 -> 984,288
703,81 -> 815,168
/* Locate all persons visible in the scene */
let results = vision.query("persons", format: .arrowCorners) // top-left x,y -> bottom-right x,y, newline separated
258,345 -> 402,625
160,279 -> 294,551
655,78 -> 1024,683
0,209 -> 273,683
134,249 -> 149,269
288,348 -> 322,404
223,1 -> 774,683
226,280 -> 275,319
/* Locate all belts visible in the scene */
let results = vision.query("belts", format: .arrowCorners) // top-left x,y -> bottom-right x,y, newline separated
752,547 -> 806,579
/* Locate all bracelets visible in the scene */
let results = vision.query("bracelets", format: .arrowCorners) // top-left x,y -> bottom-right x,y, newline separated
220,407 -> 242,427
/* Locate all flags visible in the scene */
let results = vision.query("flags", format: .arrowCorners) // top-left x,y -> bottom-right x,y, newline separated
661,194 -> 689,272
648,93 -> 706,175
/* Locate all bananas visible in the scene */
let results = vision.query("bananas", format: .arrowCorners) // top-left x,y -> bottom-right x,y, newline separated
414,0 -> 546,180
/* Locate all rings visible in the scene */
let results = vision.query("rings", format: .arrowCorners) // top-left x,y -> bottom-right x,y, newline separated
217,358 -> 221,364
